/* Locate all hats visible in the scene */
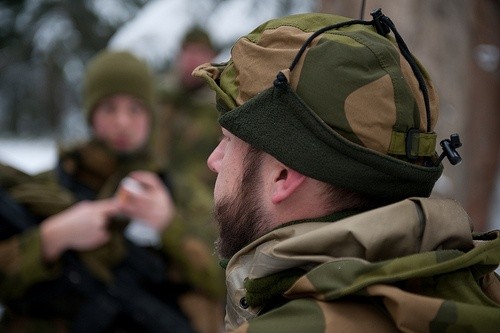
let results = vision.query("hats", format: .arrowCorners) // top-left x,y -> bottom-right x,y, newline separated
84,51 -> 154,122
190,8 -> 464,210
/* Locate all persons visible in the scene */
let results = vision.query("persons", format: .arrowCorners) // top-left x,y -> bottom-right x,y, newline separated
190,7 -> 500,333
0,29 -> 225,333
